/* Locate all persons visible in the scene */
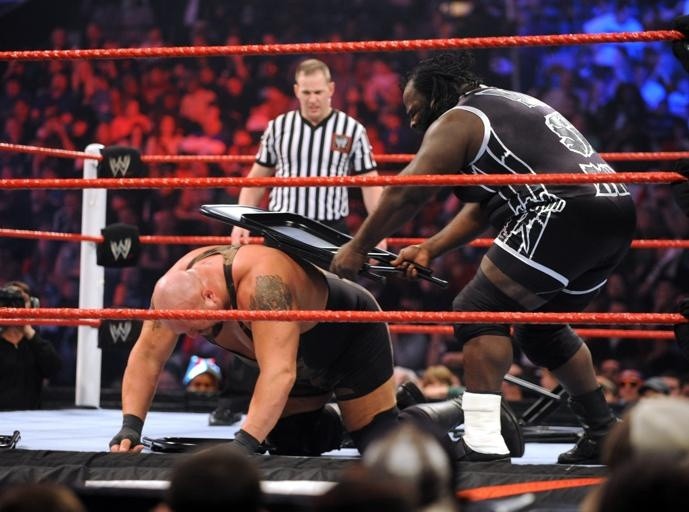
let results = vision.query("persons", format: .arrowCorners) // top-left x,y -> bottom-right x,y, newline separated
209,58 -> 382,426
328,53 -> 616,466
110,244 -> 525,456
1,389 -> 689,512
0,0 -> 689,410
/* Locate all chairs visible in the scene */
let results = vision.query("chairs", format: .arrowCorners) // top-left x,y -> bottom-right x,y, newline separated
501,373 -> 584,458
197,203 -> 448,288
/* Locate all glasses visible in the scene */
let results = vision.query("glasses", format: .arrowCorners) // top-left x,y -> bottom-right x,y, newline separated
619,382 -> 637,389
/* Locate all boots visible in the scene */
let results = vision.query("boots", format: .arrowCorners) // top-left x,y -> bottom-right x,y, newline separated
328,381 -> 523,456
559,384 -> 624,463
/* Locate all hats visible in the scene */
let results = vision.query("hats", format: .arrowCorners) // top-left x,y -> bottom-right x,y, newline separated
183,354 -> 222,386
636,378 -> 671,396
